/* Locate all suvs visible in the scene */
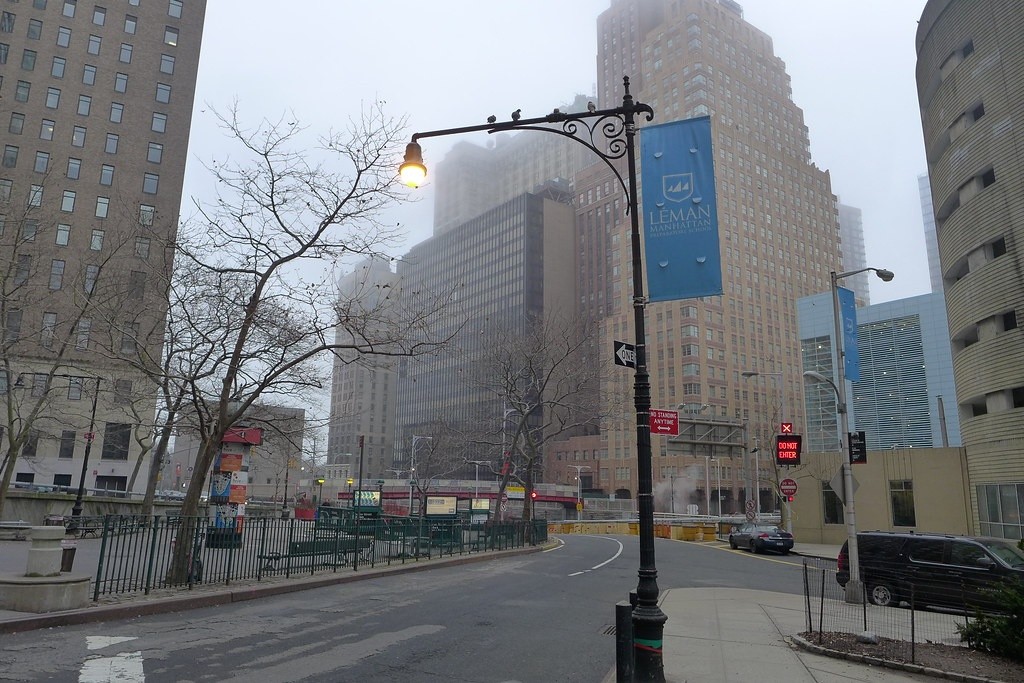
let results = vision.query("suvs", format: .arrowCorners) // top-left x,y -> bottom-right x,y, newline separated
837,529 -> 1024,616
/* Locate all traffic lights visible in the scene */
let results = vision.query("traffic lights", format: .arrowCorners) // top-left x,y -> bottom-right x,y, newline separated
787,495 -> 794,502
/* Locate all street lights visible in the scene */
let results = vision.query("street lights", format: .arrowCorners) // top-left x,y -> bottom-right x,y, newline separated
742,368 -> 792,534
532,492 -> 536,521
376,479 -> 417,517
395,102 -> 670,683
317,477 -> 325,506
408,435 -> 434,516
567,465 -> 591,521
802,267 -> 896,603
13,372 -> 102,534
752,436 -> 760,517
711,458 -> 721,518
463,460 -> 491,498
347,478 -> 353,507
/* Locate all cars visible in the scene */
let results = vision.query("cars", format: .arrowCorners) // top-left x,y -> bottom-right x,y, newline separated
729,523 -> 795,556
154,489 -> 209,502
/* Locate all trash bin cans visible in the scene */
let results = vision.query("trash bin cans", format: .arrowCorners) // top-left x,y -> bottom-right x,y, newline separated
45,517 -> 63,526
61,540 -> 77,573
533,519 -> 548,543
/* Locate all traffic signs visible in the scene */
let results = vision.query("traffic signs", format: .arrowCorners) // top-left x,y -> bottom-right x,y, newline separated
648,408 -> 679,436
613,340 -> 635,369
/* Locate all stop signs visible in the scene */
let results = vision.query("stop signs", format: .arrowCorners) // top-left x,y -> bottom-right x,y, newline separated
781,479 -> 797,495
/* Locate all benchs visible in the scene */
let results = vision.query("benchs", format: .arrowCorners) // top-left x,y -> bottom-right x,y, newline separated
257,535 -> 370,578
63,509 -> 182,539
